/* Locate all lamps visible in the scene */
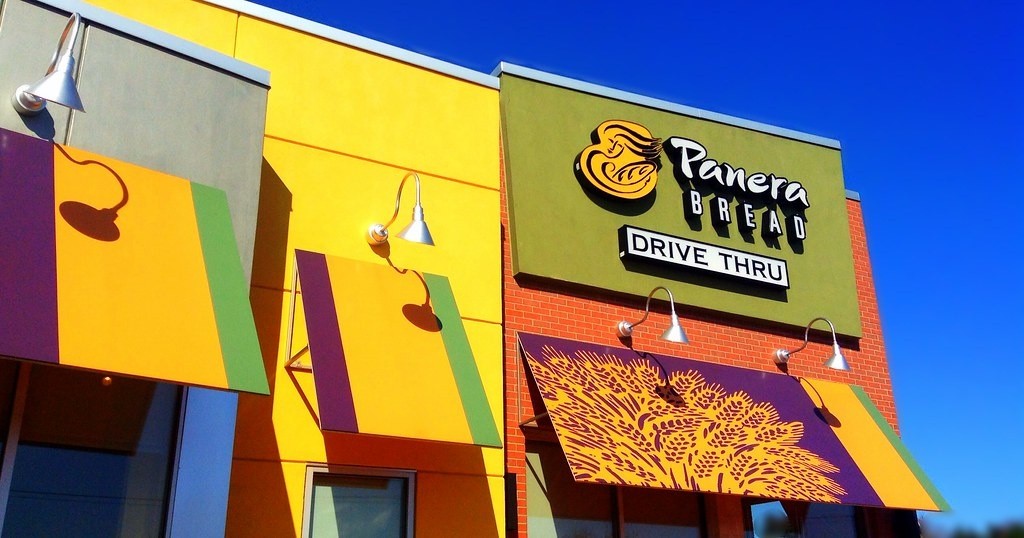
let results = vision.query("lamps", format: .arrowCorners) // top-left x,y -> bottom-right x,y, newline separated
772,317 -> 851,370
10,12 -> 87,116
615,286 -> 688,343
365,169 -> 435,247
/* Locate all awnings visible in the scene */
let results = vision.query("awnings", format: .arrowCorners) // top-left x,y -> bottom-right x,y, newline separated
518,333 -> 952,514
0,129 -> 271,398
286,250 -> 501,448
